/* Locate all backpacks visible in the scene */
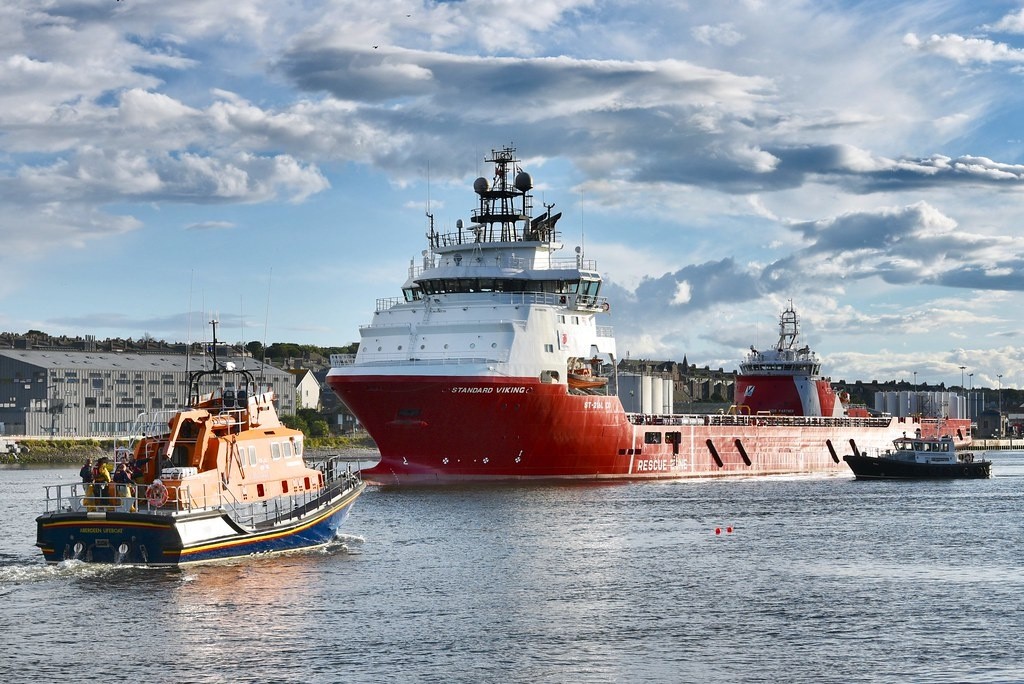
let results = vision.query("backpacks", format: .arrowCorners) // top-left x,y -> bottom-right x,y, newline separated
93,465 -> 103,478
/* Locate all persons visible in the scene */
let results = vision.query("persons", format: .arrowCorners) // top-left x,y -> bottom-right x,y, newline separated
80,453 -> 155,507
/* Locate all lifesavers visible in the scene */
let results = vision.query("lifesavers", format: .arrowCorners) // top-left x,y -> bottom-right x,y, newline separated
146,484 -> 168,507
602,302 -> 609,311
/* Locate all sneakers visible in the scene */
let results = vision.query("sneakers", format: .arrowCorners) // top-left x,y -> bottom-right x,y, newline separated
130,507 -> 136,512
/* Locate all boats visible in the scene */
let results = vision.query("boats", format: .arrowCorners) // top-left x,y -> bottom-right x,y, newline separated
843,410 -> 992,480
34,269 -> 369,568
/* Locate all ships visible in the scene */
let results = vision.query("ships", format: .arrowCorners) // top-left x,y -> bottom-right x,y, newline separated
327,142 -> 922,488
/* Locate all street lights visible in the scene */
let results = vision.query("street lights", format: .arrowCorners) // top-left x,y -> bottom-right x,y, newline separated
959,366 -> 967,418
997,375 -> 1002,414
967,374 -> 973,419
914,372 -> 918,411
